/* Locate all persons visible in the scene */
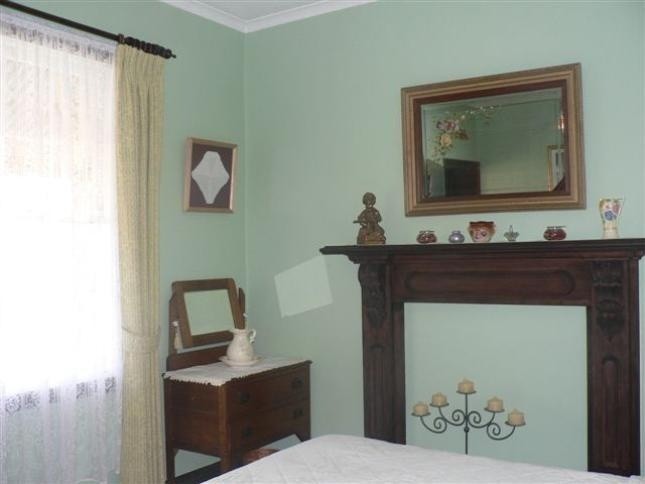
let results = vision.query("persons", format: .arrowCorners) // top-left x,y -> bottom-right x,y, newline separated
357,191 -> 386,239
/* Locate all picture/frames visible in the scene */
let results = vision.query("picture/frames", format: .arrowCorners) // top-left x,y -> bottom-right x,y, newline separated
546,142 -> 568,194
180,135 -> 240,214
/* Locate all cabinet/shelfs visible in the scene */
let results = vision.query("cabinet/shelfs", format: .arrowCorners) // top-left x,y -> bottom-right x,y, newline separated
164,355 -> 312,484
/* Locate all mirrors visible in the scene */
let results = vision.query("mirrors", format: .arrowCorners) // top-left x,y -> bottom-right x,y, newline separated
401,63 -> 586,217
168,278 -> 245,372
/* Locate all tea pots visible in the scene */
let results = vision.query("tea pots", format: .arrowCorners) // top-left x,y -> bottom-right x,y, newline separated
226,329 -> 257,363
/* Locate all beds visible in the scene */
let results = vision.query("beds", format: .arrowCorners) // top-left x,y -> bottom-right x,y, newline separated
196,432 -> 645,484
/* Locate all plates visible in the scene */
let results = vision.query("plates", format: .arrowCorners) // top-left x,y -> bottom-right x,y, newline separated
218,355 -> 259,367
